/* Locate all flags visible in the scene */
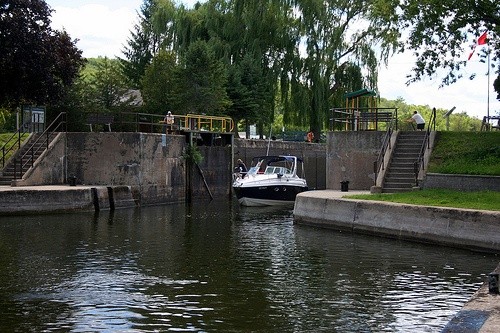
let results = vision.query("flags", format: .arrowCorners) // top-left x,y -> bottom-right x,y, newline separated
468,30 -> 489,60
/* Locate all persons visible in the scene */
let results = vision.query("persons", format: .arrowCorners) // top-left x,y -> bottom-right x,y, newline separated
164,110 -> 175,134
406,110 -> 426,131
235,158 -> 249,179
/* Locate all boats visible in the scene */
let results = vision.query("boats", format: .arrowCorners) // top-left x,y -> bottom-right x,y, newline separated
230,123 -> 308,207
